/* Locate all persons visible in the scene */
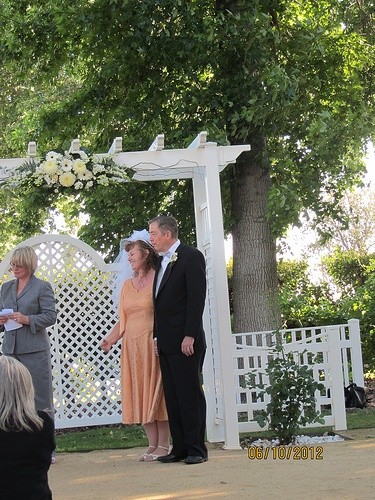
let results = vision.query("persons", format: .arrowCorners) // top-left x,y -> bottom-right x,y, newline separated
147,216 -> 210,465
100,239 -> 170,462
0,354 -> 57,500
0,245 -> 58,463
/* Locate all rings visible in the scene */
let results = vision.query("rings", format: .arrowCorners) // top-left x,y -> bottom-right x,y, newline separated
14,318 -> 17,320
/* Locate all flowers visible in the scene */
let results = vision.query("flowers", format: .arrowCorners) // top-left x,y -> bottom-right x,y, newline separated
0,147 -> 145,232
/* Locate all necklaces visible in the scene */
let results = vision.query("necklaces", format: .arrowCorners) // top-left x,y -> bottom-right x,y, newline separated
140,284 -> 146,287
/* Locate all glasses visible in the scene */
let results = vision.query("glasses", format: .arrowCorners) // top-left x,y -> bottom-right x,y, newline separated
10,261 -> 24,269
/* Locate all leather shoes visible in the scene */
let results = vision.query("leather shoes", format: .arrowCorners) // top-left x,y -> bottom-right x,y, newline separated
184,455 -> 208,464
158,454 -> 184,462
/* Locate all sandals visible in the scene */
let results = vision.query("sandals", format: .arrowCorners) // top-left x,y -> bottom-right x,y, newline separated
144,446 -> 172,461
137,446 -> 157,461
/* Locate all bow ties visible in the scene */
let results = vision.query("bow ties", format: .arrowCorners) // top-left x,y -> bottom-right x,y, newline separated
158,251 -> 172,259
